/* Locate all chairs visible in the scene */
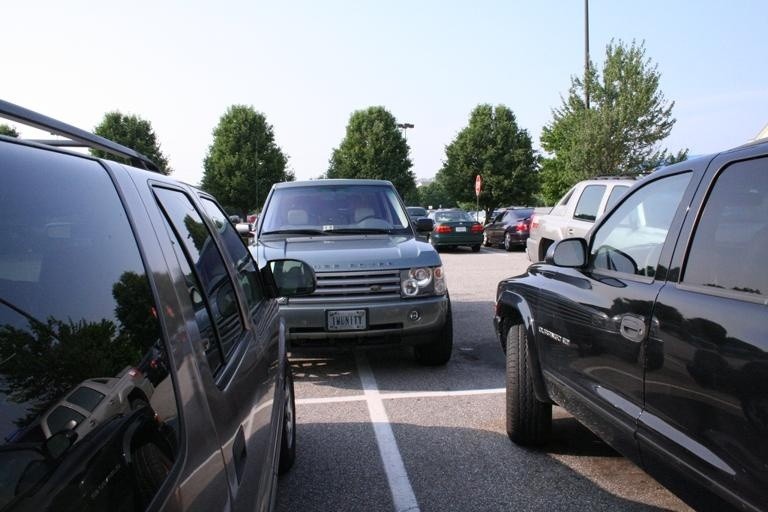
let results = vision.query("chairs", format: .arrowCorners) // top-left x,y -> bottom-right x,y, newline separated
353,205 -> 376,223
282,209 -> 310,229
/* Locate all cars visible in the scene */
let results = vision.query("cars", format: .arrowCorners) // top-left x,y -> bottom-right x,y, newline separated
492,140 -> 767,512
525,176 -> 672,273
405,205 -> 536,253
1,99 -> 318,512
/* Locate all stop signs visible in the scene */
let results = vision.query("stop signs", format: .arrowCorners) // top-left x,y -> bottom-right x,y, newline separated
475,175 -> 482,196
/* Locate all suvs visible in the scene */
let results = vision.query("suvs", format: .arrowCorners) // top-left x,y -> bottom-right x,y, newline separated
232,176 -> 452,365
